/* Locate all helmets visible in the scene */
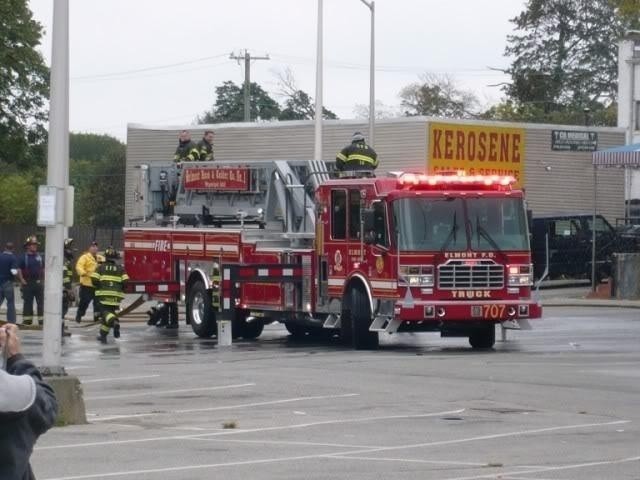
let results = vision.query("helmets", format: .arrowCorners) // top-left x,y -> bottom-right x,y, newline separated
352,132 -> 364,142
64,238 -> 78,253
104,245 -> 119,260
24,236 -> 41,247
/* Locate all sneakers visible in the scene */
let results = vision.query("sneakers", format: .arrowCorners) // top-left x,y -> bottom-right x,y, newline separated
157,321 -> 180,329
95,332 -> 108,344
112,320 -> 121,338
74,315 -> 99,324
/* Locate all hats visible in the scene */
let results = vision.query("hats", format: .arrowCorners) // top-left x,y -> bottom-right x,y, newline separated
90,240 -> 97,247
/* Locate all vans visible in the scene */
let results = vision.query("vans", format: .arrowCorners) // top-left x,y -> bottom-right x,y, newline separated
525,210 -> 627,280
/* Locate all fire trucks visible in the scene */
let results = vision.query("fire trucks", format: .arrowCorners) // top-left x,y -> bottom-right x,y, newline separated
123,158 -> 542,349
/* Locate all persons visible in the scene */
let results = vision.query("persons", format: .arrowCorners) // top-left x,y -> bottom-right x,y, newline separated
75,241 -> 100,324
197,130 -> 216,161
335,131 -> 379,179
18,235 -> 44,326
147,300 -> 170,327
172,131 -> 199,181
0,242 -> 18,323
90,246 -> 129,343
61,238 -> 76,336
0,321 -> 57,480
